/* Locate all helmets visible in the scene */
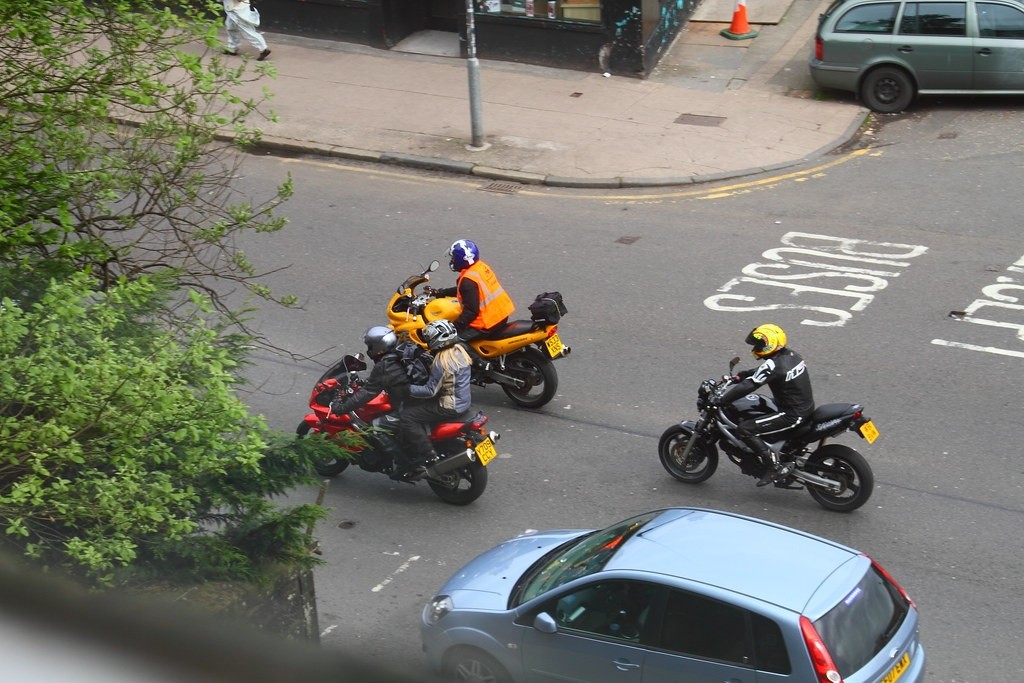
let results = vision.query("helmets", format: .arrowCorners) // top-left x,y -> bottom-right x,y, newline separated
448,239 -> 479,272
422,320 -> 458,350
751,324 -> 787,360
365,325 -> 397,360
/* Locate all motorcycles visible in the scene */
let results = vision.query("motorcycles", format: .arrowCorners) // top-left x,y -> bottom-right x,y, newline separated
388,259 -> 571,410
295,353 -> 501,503
656,359 -> 879,512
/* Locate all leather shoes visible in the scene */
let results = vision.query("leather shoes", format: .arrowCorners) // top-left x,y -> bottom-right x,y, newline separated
222,50 -> 238,56
256,49 -> 271,62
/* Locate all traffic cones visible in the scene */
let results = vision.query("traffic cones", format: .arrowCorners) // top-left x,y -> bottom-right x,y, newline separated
719,0 -> 760,41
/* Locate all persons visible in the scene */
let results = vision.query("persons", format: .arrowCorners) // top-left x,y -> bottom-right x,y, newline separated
708,324 -> 814,486
221,0 -> 270,60
331,326 -> 429,480
391,318 -> 472,465
425,239 -> 515,371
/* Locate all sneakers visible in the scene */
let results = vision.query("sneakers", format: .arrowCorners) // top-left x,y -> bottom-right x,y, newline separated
756,461 -> 789,487
390,450 -> 440,479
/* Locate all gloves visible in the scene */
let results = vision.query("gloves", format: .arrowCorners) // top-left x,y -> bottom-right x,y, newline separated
710,397 -> 720,406
331,403 -> 348,415
436,288 -> 446,298
420,353 -> 434,362
736,371 -> 747,383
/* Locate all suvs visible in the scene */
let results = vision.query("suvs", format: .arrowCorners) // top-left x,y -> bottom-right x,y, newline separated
422,506 -> 928,683
810,1 -> 1024,112
